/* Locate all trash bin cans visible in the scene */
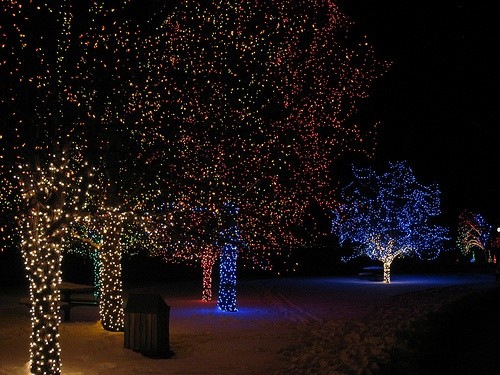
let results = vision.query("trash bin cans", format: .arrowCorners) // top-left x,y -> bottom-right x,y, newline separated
123,292 -> 171,356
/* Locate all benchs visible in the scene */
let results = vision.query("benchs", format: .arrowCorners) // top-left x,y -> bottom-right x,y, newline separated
18,282 -> 100,322
358,265 -> 383,280
